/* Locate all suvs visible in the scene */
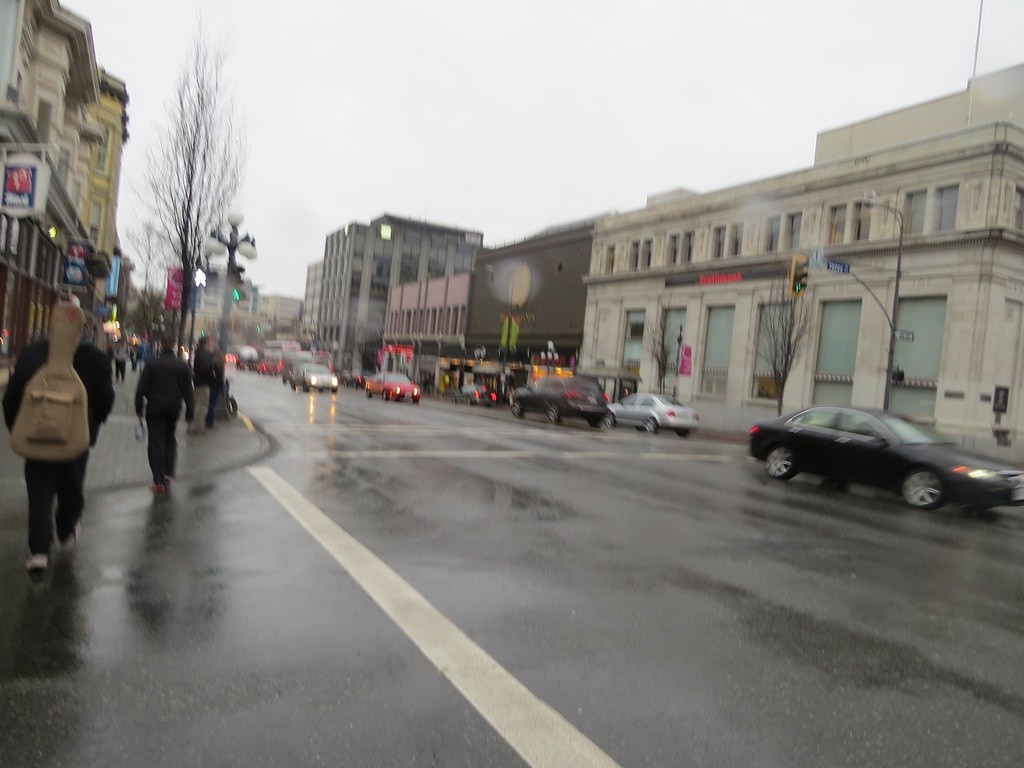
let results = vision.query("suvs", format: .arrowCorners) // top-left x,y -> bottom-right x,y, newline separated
508,374 -> 608,428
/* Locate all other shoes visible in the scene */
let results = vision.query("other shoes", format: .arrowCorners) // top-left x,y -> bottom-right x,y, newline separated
150,484 -> 164,492
59,519 -> 81,551
25,554 -> 48,570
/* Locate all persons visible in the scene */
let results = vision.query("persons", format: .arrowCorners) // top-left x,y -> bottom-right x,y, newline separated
135,347 -> 195,494
107,346 -> 113,358
129,345 -> 145,374
2,304 -> 116,571
115,347 -> 126,381
197,335 -> 223,428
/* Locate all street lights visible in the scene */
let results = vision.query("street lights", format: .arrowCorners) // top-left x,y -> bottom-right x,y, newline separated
203,209 -> 259,422
863,202 -> 904,414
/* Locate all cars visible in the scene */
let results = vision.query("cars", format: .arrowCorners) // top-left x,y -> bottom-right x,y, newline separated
603,392 -> 700,438
450,384 -> 497,409
290,363 -> 339,393
747,403 -> 1024,518
365,371 -> 421,405
223,340 -> 372,390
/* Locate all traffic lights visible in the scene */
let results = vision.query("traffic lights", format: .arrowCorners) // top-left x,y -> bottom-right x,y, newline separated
789,255 -> 810,297
